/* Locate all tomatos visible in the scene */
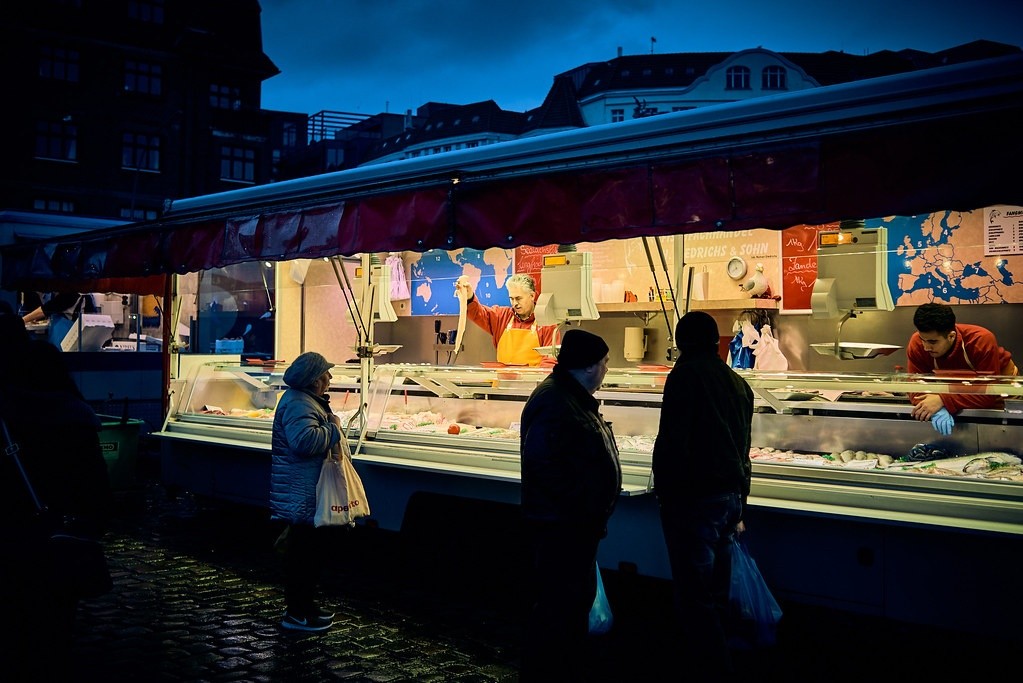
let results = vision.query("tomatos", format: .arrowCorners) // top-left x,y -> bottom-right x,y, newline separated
448,423 -> 460,435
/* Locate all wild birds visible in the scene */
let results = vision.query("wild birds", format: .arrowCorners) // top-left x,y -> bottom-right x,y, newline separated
740,262 -> 768,298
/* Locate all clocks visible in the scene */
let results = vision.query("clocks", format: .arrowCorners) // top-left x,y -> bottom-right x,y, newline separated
726,256 -> 748,281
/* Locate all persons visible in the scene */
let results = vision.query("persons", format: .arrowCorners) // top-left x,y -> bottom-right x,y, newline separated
651,312 -> 754,683
23,290 -> 96,351
522,331 -> 621,683
907,304 -> 1023,435
725,310 -> 788,370
457,273 -> 560,380
268,353 -> 352,631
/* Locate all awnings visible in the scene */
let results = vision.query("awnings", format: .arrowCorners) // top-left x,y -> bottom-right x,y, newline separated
33,57 -> 1023,346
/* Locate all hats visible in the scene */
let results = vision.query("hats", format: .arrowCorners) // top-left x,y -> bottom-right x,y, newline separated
675,311 -> 719,351
282,353 -> 334,389
556,329 -> 608,369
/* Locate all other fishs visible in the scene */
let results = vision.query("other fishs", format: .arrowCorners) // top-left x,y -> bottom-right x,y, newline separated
878,454 -> 893,467
854,451 -> 866,460
750,446 -> 819,463
867,453 -> 878,463
617,437 -> 653,451
903,452 -> 1023,484
840,450 -> 855,462
449,275 -> 469,364
830,452 -> 845,465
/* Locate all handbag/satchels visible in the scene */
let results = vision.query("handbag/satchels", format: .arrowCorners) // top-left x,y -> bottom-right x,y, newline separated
588,560 -> 613,633
313,422 -> 371,528
728,539 -> 782,623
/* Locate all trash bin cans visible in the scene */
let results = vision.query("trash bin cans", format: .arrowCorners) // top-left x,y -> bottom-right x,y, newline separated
95,412 -> 145,467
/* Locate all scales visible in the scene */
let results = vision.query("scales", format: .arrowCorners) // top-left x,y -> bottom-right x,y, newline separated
532,243 -> 601,361
346,252 -> 404,355
809,219 -> 904,361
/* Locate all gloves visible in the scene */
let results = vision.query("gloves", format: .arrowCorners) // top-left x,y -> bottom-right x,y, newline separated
930,406 -> 954,435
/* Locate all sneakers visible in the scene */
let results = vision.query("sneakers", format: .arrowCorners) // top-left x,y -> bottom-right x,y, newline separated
282,611 -> 334,630
313,604 -> 336,620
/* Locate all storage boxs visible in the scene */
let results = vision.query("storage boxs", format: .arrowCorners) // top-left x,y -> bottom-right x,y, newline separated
59,312 -> 117,352
111,340 -> 159,352
